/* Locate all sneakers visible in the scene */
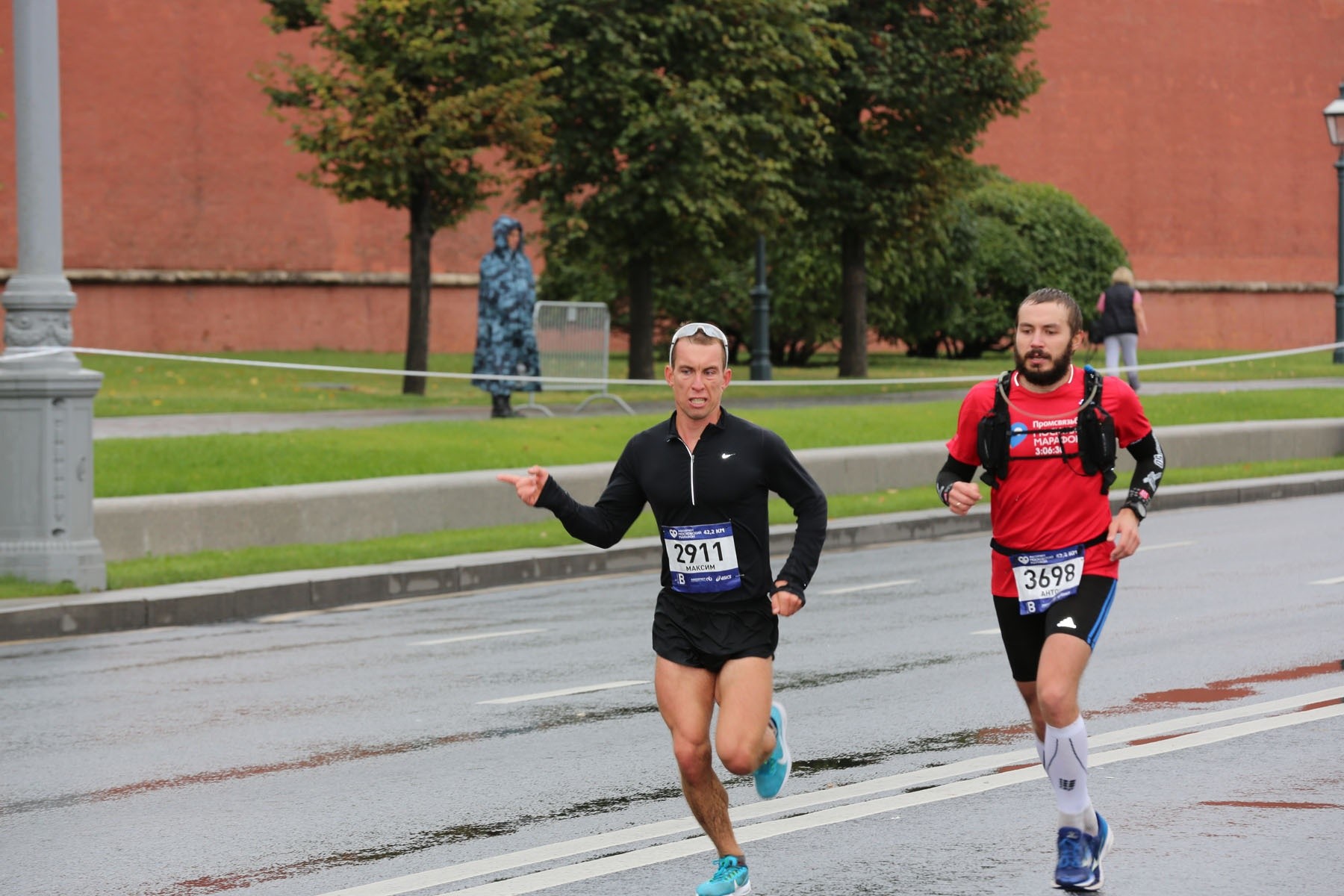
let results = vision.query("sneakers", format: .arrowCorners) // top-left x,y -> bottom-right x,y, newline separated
696,855 -> 751,896
1054,826 -> 1095,887
1051,813 -> 1114,892
754,700 -> 792,799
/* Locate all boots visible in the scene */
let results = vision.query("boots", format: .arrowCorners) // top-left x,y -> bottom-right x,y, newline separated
491,394 -> 527,417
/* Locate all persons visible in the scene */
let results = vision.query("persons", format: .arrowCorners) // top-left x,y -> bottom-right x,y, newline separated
1090,264 -> 1146,389
934,288 -> 1167,891
495,321 -> 828,896
471,215 -> 544,419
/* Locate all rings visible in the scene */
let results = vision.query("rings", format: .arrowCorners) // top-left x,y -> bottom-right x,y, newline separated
955,502 -> 961,507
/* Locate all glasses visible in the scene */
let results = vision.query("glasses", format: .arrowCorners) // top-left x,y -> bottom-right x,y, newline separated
669,322 -> 729,370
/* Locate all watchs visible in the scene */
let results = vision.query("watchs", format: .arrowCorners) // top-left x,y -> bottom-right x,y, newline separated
1120,501 -> 1147,522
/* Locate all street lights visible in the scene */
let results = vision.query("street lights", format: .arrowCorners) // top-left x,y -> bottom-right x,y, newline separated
1323,81 -> 1344,366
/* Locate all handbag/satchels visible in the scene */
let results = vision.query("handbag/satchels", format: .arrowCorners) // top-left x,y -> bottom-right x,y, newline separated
1087,311 -> 1106,344
